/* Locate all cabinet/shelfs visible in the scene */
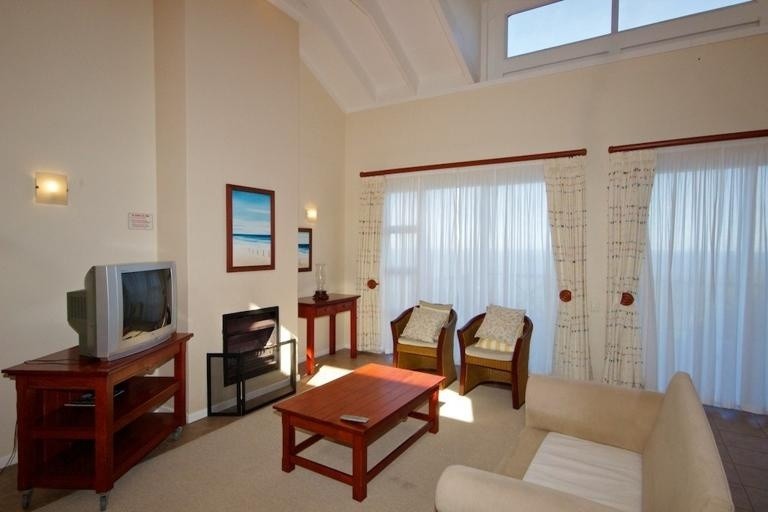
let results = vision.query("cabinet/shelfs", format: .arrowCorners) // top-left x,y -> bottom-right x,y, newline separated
2,328 -> 195,511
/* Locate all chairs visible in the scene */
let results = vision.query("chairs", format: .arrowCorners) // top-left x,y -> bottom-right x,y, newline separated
457,311 -> 533,413
388,303 -> 457,392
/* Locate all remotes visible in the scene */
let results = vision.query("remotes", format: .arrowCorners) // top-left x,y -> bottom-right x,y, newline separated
340,414 -> 370,424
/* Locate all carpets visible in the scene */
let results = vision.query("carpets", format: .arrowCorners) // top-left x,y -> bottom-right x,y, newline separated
34,376 -> 527,511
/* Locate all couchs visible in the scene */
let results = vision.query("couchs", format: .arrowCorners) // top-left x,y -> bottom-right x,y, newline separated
429,368 -> 737,511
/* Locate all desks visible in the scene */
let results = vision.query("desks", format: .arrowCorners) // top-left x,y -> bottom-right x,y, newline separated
298,291 -> 361,376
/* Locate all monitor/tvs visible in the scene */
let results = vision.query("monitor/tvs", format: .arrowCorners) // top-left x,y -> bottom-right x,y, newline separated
67,261 -> 178,362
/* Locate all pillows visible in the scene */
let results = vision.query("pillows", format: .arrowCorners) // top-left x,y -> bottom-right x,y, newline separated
474,303 -> 527,345
471,317 -> 524,351
418,300 -> 452,329
397,304 -> 450,344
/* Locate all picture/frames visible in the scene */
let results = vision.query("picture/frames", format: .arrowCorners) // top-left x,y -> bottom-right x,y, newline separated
224,182 -> 276,274
297,227 -> 313,273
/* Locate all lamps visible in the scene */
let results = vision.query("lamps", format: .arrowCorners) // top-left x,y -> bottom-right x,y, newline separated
34,170 -> 71,207
